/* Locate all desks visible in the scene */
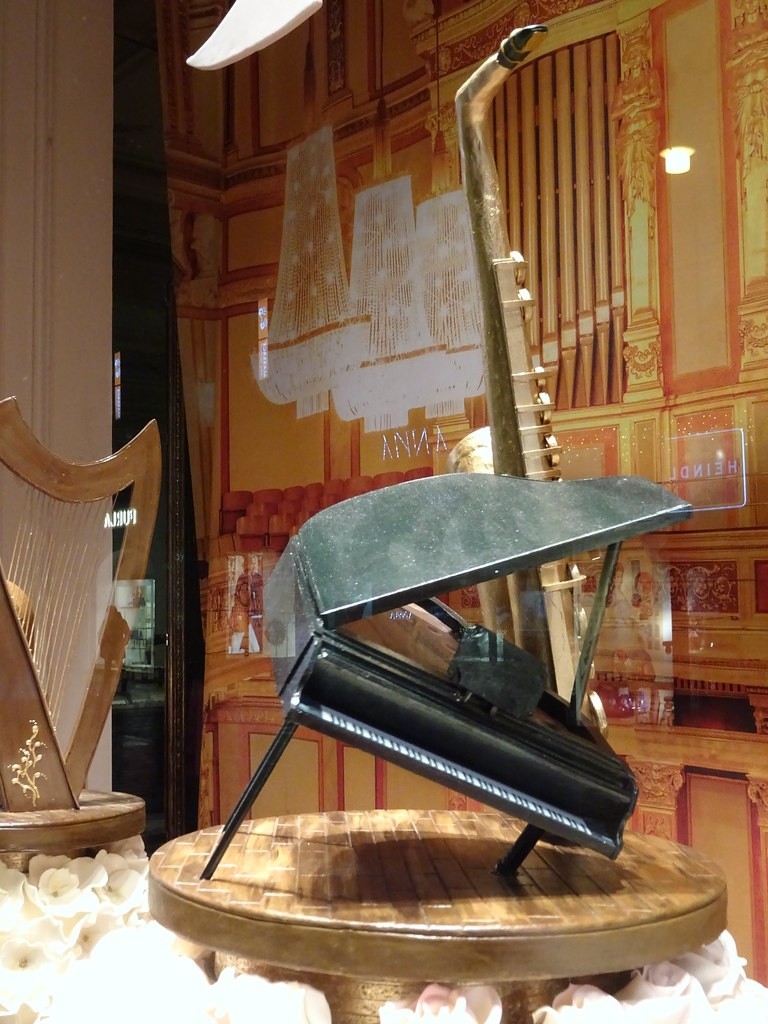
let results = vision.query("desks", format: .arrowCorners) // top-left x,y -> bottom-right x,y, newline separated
0,789 -> 147,875
148,807 -> 730,1024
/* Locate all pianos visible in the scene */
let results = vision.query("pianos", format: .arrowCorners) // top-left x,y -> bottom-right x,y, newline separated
197,470 -> 692,882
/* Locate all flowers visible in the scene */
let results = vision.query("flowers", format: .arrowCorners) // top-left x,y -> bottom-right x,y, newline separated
0,834 -> 746,1024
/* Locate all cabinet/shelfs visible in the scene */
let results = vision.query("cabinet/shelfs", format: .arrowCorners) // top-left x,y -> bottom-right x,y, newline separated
113,579 -> 154,673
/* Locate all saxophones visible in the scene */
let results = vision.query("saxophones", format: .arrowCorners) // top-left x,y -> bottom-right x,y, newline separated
444,20 -> 605,739
